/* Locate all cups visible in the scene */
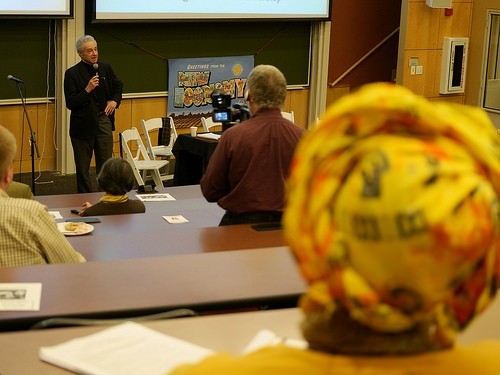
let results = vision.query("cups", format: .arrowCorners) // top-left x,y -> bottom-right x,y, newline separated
190,127 -> 197,137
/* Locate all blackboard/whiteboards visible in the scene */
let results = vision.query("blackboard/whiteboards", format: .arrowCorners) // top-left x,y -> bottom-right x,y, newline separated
84,0 -> 313,99
0,14 -> 58,106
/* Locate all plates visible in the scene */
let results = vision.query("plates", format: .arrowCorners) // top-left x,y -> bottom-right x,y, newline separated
57,221 -> 94,235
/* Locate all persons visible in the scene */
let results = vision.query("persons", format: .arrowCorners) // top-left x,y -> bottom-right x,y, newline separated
0,127 -> 88,269
64,35 -> 123,193
168,82 -> 500,375
78,157 -> 146,217
200,64 -> 310,226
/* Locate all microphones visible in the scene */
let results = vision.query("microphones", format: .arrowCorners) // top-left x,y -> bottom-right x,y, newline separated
93,63 -> 98,82
8,75 -> 24,83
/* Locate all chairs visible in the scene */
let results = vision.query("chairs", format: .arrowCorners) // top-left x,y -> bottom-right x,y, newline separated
120,110 -> 299,191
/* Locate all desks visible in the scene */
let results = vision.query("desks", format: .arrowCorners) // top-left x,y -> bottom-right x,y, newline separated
0,187 -> 309,375
170,131 -> 223,186
